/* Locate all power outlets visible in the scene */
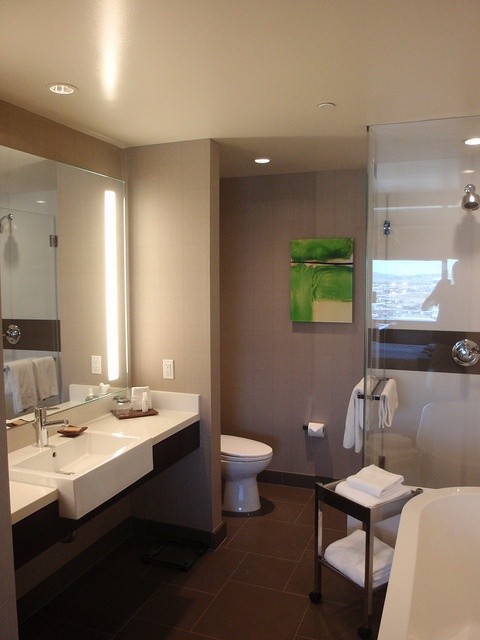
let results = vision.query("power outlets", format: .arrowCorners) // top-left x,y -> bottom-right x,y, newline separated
162,359 -> 174,380
91,356 -> 101,375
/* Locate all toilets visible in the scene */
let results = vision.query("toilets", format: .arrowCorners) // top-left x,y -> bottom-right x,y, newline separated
221,435 -> 273,513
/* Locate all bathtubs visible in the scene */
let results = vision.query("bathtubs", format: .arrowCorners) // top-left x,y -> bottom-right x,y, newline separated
378,487 -> 480,640
415,398 -> 479,467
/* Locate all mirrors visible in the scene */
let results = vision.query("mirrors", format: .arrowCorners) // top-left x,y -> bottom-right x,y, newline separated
0,144 -> 129,432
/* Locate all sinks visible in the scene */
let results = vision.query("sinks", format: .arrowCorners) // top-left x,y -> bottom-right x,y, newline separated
10,430 -> 139,481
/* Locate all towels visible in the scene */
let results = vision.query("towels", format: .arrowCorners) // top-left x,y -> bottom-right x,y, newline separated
324,529 -> 395,589
343,375 -> 374,454
378,379 -> 399,429
334,479 -> 412,509
346,464 -> 404,498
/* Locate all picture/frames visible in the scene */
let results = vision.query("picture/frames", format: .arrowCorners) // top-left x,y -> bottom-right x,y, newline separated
290,236 -> 354,324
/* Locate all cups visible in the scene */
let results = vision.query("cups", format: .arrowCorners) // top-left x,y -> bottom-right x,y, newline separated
117,398 -> 131,416
111,395 -> 127,414
86,395 -> 99,404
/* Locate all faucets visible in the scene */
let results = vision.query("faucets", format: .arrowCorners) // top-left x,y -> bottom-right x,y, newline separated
34,406 -> 69,447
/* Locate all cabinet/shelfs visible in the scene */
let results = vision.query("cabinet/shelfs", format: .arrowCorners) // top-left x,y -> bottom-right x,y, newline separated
309,467 -> 422,638
